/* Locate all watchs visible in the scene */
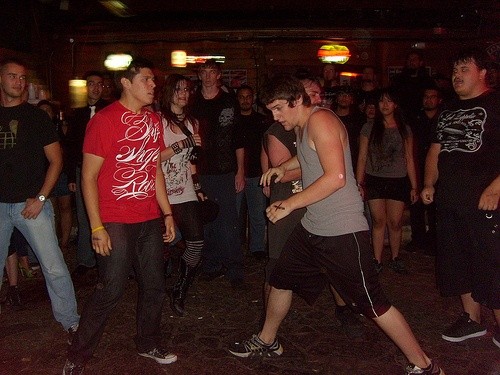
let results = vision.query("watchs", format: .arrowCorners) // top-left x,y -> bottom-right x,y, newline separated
37,194 -> 46,201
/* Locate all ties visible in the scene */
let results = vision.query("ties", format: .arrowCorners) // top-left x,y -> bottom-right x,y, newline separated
89,106 -> 96,119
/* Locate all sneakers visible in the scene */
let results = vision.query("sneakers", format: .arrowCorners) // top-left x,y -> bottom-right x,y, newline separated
493,333 -> 500,349
66,324 -> 80,345
62,357 -> 84,375
9,285 -> 24,306
406,358 -> 445,375
228,331 -> 283,358
138,348 -> 177,365
442,312 -> 487,342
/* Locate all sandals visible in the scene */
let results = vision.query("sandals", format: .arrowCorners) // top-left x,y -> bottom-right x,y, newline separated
71,266 -> 97,283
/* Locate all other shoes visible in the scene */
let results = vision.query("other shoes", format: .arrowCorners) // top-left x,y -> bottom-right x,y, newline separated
389,257 -> 410,274
335,306 -> 362,337
199,271 -> 223,281
372,258 -> 383,273
228,272 -> 243,288
405,240 -> 426,252
254,251 -> 267,264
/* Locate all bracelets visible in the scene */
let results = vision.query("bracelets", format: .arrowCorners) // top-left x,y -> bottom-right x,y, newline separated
172,143 -> 182,154
92,226 -> 104,232
165,214 -> 173,217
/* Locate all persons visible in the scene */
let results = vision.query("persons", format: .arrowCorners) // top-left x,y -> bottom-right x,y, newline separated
62,62 -> 178,375
420,50 -> 500,346
236,85 -> 268,271
190,61 -> 246,277
64,70 -> 110,279
262,77 -> 365,324
161,73 -> 208,316
229,73 -> 446,375
321,50 -> 445,271
0,57 -> 80,344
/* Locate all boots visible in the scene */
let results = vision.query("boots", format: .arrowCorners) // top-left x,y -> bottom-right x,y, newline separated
169,256 -> 196,315
